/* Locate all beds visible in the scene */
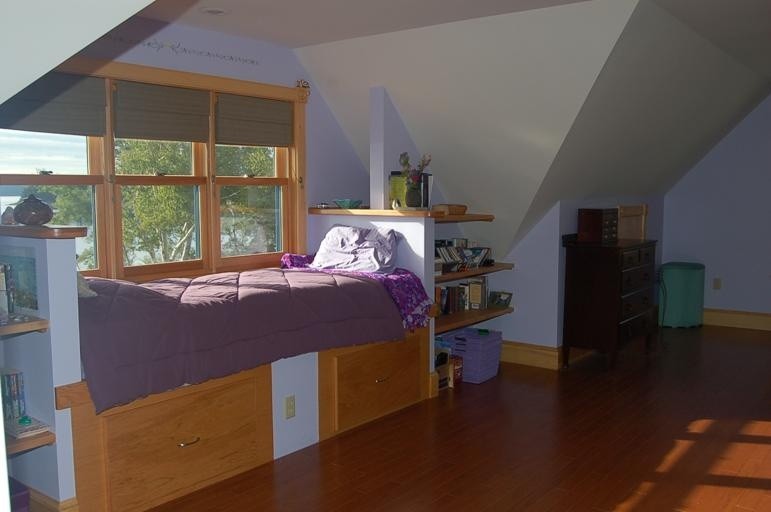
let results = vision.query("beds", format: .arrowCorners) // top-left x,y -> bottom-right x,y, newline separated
79,265 -> 430,512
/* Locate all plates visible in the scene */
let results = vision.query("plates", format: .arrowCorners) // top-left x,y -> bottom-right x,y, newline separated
333,200 -> 362,208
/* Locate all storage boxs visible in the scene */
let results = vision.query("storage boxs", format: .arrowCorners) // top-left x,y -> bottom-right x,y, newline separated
435,328 -> 503,390
579,208 -> 618,242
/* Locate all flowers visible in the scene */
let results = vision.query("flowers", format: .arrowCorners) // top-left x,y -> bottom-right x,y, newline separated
398,152 -> 433,190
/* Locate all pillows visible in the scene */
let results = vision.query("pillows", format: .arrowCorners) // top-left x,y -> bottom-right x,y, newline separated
309,222 -> 400,277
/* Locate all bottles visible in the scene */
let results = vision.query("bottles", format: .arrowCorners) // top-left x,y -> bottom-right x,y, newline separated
392,199 -> 401,209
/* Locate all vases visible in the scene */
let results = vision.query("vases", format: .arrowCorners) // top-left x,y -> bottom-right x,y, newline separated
405,187 -> 422,208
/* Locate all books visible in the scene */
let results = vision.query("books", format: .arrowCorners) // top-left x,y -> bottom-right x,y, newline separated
389,171 -> 467,215
436,239 -> 489,320
0,262 -> 15,326
0,368 -> 50,440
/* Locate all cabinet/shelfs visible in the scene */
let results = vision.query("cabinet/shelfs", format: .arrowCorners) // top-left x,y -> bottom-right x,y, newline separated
561,234 -> 658,373
0,313 -> 56,457
73,362 -> 274,512
319,328 -> 429,440
435,214 -> 514,337
